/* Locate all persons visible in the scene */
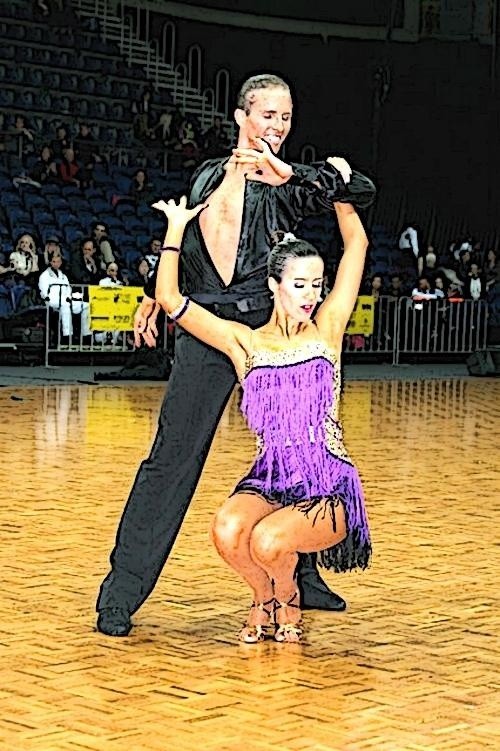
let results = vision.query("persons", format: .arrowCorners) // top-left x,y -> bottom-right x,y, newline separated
147,154 -> 372,645
94,72 -> 377,637
2,92 -> 500,368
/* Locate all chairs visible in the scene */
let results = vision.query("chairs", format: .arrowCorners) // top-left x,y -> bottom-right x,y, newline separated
0,0 -> 431,349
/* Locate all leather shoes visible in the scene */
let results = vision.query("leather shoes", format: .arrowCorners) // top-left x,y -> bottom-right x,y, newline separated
95,607 -> 134,638
299,576 -> 346,614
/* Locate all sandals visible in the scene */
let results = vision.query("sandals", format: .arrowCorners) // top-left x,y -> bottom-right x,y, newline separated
274,592 -> 307,645
236,596 -> 274,644
